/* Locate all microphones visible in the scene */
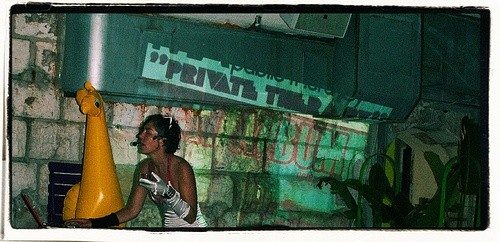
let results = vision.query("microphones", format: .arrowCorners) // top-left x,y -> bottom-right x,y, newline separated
132,142 -> 139,146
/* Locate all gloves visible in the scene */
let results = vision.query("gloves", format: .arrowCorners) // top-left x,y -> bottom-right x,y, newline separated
138,172 -> 190,219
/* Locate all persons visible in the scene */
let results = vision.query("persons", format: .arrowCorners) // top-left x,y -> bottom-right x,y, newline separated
62,113 -> 209,228
